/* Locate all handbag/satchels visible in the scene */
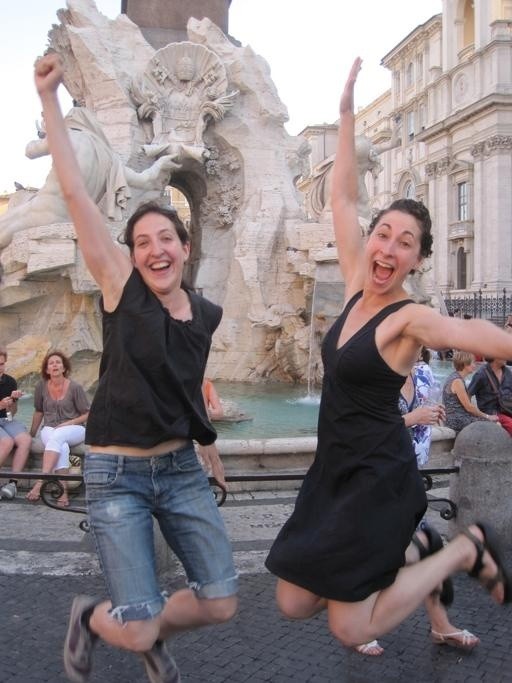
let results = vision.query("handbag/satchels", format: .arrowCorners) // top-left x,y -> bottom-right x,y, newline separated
499,395 -> 512,417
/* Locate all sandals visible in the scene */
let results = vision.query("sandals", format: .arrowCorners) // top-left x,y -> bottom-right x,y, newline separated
431,629 -> 480,647
461,520 -> 510,605
412,527 -> 453,604
355,639 -> 384,656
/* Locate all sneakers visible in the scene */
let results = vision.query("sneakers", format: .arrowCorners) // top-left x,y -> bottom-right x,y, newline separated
1,482 -> 17,499
63,594 -> 104,683
143,640 -> 179,683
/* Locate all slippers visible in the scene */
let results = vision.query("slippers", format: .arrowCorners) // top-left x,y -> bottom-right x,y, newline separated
25,491 -> 40,502
56,494 -> 70,507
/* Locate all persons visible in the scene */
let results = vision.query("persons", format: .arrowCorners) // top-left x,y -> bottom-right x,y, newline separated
0,352 -> 33,500
25,352 -> 91,506
355,309 -> 512,657
265,57 -> 512,648
200,379 -> 223,421
33,54 -> 240,683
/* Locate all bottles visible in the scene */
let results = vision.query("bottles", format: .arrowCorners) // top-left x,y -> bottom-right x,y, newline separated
426,382 -> 441,408
